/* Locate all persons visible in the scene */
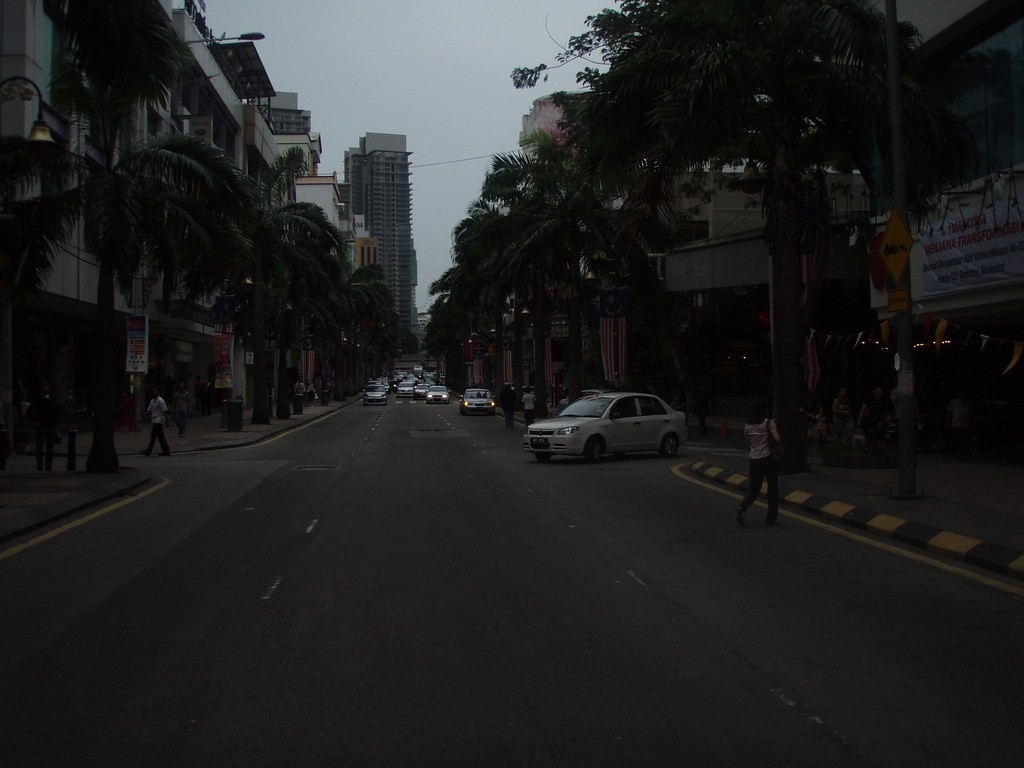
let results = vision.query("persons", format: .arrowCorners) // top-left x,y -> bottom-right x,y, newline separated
26,383 -> 57,472
295,378 -> 315,406
696,382 -> 714,436
831,386 -> 850,445
192,376 -> 206,411
521,387 -> 537,426
139,386 -> 170,456
500,385 -> 516,429
735,400 -> 782,528
857,384 -> 893,455
171,383 -> 192,437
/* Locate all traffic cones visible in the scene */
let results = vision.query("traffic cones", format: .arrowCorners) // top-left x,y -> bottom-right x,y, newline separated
718,416 -> 731,439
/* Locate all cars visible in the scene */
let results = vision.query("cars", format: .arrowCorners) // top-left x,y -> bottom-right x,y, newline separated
522,394 -> 689,461
363,380 -> 387,405
385,369 -> 438,400
915,400 -> 1024,454
425,386 -> 451,404
459,388 -> 495,416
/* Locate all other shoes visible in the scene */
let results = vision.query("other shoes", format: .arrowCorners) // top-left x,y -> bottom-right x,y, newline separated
767,519 -> 778,525
159,453 -> 170,456
736,507 -> 745,526
178,433 -> 185,437
139,450 -> 149,456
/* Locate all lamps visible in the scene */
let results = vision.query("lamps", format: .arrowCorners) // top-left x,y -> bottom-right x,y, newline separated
183,32 -> 264,43
0,74 -> 55,151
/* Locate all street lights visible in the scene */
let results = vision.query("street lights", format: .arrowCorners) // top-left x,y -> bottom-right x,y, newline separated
739,128 -> 809,471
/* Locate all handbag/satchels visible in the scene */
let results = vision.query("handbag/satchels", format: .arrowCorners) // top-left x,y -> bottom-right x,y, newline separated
766,418 -> 786,456
54,433 -> 62,443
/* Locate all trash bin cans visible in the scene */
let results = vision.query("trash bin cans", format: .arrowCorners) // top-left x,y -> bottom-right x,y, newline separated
227,400 -> 243,429
320,390 -> 329,405
293,394 -> 304,414
